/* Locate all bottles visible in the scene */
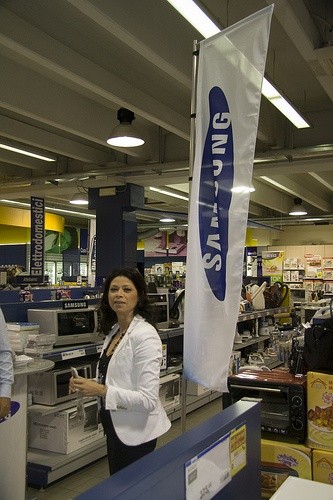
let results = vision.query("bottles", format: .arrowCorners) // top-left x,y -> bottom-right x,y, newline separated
168,271 -> 181,287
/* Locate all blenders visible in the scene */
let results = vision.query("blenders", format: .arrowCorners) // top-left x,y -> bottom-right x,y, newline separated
258,315 -> 277,337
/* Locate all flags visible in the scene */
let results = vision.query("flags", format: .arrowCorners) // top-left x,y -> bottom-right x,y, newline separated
180,8 -> 272,394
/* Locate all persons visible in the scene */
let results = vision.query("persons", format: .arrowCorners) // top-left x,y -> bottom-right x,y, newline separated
69,266 -> 172,477
0,308 -> 17,419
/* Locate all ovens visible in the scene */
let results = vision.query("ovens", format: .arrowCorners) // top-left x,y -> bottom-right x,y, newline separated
26,306 -> 105,346
127,292 -> 173,330
227,352 -> 243,376
28,399 -> 105,454
26,362 -> 96,406
156,373 -> 183,410
185,375 -> 212,396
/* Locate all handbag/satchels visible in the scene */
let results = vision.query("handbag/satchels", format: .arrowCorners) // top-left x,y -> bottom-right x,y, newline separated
288,339 -> 305,375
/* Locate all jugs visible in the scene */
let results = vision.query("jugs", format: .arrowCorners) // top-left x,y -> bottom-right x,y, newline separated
248,281 -> 288,309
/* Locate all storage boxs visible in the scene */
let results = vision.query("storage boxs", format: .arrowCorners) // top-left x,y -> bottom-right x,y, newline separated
28,400 -> 103,456
261,440 -> 313,481
283,258 -> 333,294
186,380 -> 211,396
312,449 -> 333,486
228,351 -> 241,376
158,373 -> 182,411
160,344 -> 168,372
307,371 -> 333,452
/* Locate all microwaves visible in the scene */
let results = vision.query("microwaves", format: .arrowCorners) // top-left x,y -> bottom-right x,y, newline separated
222,367 -> 307,444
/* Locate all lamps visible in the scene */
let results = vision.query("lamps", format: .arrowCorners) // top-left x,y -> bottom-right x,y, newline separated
0,137 -> 57,162
288,198 -> 308,216
167,0 -> 315,130
149,185 -> 190,202
159,214 -> 176,222
106,107 -> 145,148
69,193 -> 89,205
259,174 -> 331,212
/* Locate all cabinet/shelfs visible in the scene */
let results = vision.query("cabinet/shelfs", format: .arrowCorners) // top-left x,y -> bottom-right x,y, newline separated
26,306 -> 290,491
283,268 -> 305,291
322,267 -> 333,295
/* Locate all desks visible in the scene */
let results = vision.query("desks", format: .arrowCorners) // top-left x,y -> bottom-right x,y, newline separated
0,358 -> 55,500
